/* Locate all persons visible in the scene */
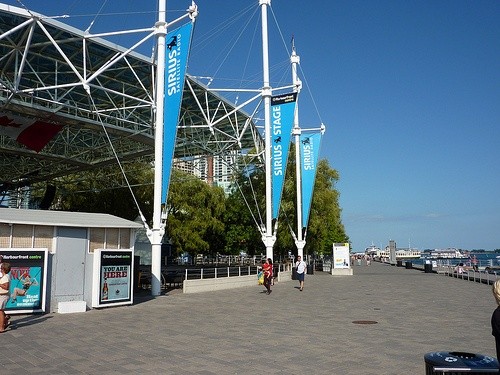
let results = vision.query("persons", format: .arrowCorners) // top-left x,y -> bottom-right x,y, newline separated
453,255 -> 495,276
491,280 -> 500,364
0,255 -> 10,321
258,258 -> 273,295
11,272 -> 38,304
0,262 -> 11,333
295,256 -> 306,290
350,252 -> 374,266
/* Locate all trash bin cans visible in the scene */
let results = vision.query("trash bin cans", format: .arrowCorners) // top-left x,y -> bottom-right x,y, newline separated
424,350 -> 499,375
424,264 -> 432,273
405,262 -> 412,269
397,261 -> 402,266
